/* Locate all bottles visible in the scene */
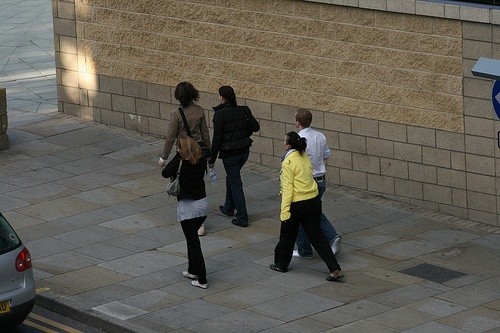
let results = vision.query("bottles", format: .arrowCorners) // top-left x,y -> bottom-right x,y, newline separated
209,168 -> 217,185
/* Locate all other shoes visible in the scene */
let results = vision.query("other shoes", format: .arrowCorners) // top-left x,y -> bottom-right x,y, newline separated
198,222 -> 205,235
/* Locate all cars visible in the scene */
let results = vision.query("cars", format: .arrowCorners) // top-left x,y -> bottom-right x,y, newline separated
0,213 -> 37,333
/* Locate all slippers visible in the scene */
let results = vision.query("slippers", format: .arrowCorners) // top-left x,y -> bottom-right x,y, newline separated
270,264 -> 288,273
326,275 -> 344,281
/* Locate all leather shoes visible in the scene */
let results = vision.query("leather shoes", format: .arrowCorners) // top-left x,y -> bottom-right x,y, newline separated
219,205 -> 233,216
232,219 -> 248,227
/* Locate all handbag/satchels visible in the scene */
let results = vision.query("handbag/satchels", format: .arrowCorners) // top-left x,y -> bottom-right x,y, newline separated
167,159 -> 183,197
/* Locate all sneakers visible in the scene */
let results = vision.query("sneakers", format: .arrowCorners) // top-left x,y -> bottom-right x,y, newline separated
183,271 -> 208,289
331,237 -> 341,255
292,250 -> 313,259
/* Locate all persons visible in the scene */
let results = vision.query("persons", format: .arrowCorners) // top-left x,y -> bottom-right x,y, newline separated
158,81 -> 209,235
161,132 -> 208,287
207,85 -> 260,226
269,130 -> 345,281
292,107 -> 342,259
